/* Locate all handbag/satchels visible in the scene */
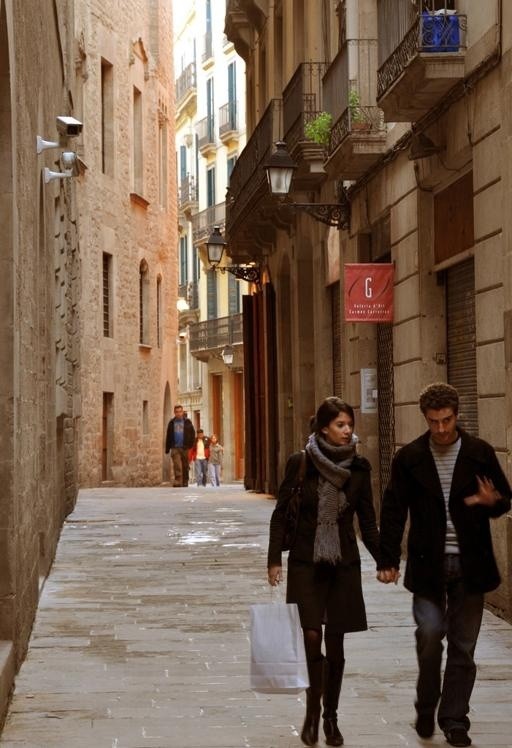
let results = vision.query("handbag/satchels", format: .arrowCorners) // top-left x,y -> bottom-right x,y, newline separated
280,450 -> 307,551
250,579 -> 310,695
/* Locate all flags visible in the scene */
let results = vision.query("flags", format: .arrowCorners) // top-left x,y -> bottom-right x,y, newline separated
342,262 -> 396,324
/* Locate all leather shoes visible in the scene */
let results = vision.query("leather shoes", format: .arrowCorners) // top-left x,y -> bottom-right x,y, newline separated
416,716 -> 472,747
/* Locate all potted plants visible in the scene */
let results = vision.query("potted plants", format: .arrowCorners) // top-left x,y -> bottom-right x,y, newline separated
349,91 -> 371,135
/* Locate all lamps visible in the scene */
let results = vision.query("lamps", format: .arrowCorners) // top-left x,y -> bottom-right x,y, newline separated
204,227 -> 262,293
263,137 -> 350,235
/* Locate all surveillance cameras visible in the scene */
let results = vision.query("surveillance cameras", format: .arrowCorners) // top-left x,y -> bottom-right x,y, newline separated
56,116 -> 84,139
61,151 -> 80,178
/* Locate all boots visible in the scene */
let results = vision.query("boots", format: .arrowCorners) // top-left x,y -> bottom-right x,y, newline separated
301,687 -> 344,746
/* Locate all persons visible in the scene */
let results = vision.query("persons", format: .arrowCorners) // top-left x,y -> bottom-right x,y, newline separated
189,428 -> 212,488
165,404 -> 196,487
266,396 -> 403,747
375,380 -> 512,747
207,433 -> 224,487
